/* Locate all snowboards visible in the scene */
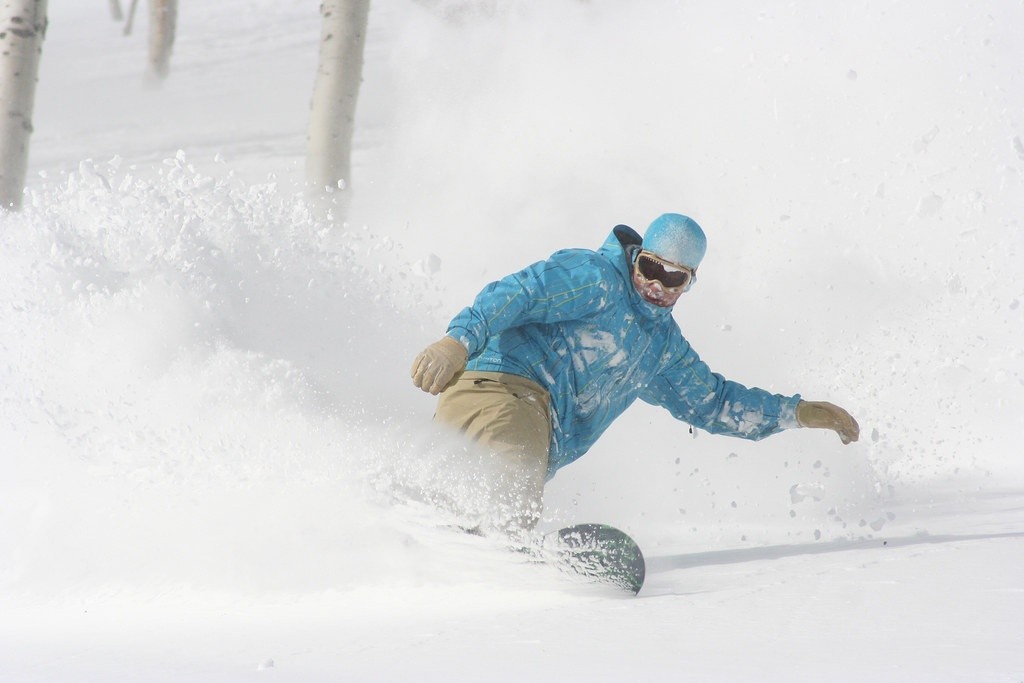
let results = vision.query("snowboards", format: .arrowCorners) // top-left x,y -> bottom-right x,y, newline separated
512,523 -> 647,602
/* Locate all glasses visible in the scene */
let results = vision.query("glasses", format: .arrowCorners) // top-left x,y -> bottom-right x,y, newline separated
635,254 -> 691,294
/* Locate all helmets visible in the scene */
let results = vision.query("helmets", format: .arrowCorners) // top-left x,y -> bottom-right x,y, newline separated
640,213 -> 707,278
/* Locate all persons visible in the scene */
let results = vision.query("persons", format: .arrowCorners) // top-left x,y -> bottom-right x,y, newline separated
410,214 -> 860,554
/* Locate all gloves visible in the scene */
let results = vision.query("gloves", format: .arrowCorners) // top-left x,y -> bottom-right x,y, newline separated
796,400 -> 860,445
410,337 -> 468,395
